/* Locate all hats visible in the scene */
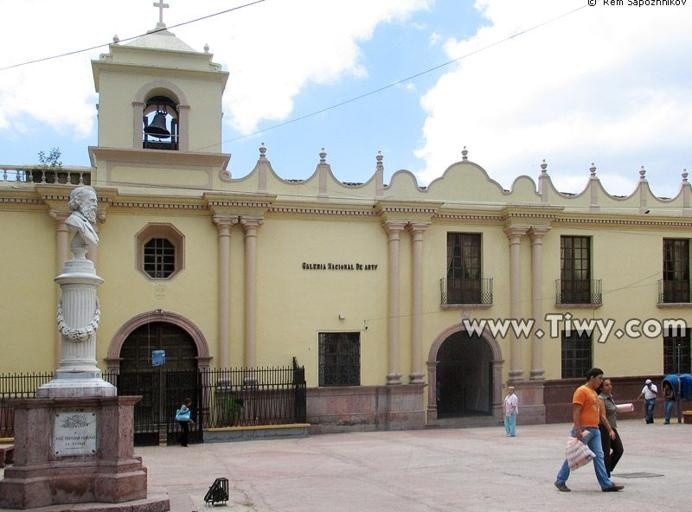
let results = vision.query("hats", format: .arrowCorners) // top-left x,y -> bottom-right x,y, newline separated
646,379 -> 651,384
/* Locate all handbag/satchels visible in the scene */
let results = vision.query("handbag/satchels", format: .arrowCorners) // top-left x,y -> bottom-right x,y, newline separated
175,409 -> 191,422
566,437 -> 596,471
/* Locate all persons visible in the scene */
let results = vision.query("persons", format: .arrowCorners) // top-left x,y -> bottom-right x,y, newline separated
64,185 -> 100,246
637,378 -> 659,424
553,366 -> 626,492
502,385 -> 520,437
662,383 -> 674,424
596,377 -> 623,478
174,397 -> 195,447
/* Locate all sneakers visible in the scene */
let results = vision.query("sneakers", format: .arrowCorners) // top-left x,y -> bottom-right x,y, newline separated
602,483 -> 624,492
646,418 -> 670,425
554,481 -> 572,492
